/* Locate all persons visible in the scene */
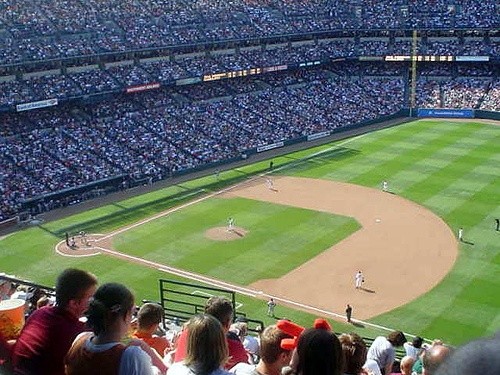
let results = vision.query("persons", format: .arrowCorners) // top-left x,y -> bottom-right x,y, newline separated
291,328 -> 343,375
459,228 -> 463,241
79,230 -> 86,242
65,232 -> 69,245
495,218 -> 500,231
337,332 -> 368,375
174,296 -> 251,373
61,282 -> 155,375
7,268 -> 98,375
233,317 -> 259,354
227,217 -> 234,232
268,298 -> 277,318
1,276 -> 179,343
355,270 -> 364,289
228,322 -> 297,375
131,303 -> 169,364
167,313 -> 238,375
346,304 -> 352,322
228,322 -> 260,364
69,237 -> 77,247
364,330 -> 408,375
382,336 -> 500,374
0,0 -> 499,219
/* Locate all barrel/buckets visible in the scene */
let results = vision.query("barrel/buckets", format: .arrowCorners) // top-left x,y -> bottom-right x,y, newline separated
0,299 -> 26,339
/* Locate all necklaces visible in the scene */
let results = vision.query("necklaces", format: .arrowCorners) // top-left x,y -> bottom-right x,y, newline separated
254,365 -> 265,375
